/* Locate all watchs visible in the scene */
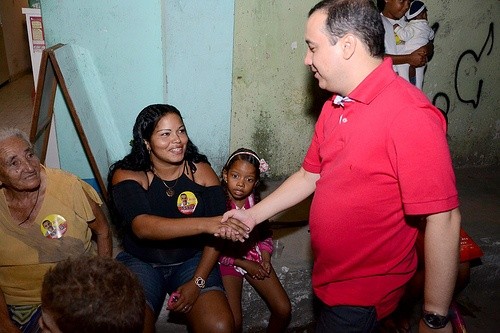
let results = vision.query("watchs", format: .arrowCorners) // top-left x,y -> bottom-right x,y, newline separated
423,304 -> 450,329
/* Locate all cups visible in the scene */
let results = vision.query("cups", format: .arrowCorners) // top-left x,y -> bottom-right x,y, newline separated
271,240 -> 284,259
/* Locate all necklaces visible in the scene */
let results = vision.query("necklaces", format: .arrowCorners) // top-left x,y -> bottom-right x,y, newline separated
151,162 -> 181,197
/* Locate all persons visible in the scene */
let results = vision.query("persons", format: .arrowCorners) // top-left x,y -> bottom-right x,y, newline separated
215,0 -> 463,333
393,0 -> 435,92
218,148 -> 292,333
0,128 -> 114,333
376,0 -> 435,92
37,257 -> 156,333
108,104 -> 250,333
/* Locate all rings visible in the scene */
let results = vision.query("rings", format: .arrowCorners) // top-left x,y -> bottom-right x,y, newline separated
184,306 -> 188,311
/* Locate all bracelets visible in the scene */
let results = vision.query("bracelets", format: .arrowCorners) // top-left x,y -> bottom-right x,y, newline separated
193,276 -> 205,288
